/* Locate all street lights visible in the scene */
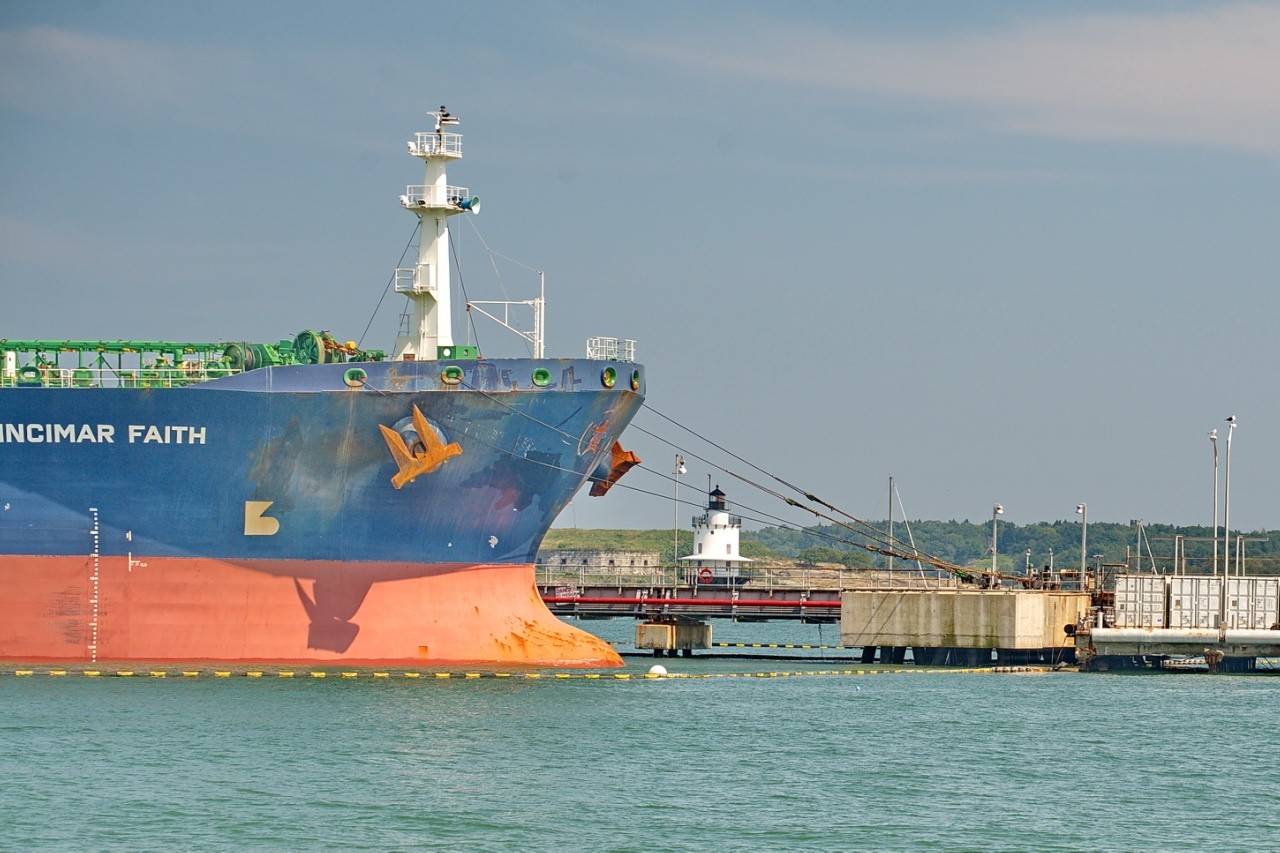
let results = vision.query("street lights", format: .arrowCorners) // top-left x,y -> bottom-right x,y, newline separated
1048,548 -> 1053,578
1174,535 -> 1184,576
989,502 -> 1005,586
1224,414 -> 1238,623
1075,503 -> 1087,591
1235,536 -> 1244,577
1092,554 -> 1104,598
1208,435 -> 1220,576
1025,549 -> 1031,575
671,455 -> 687,599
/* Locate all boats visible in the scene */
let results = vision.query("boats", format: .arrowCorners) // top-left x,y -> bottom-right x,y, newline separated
0,106 -> 651,673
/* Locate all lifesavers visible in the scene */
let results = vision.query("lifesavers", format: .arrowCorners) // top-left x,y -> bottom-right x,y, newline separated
17,366 -> 41,384
699,569 -> 714,583
